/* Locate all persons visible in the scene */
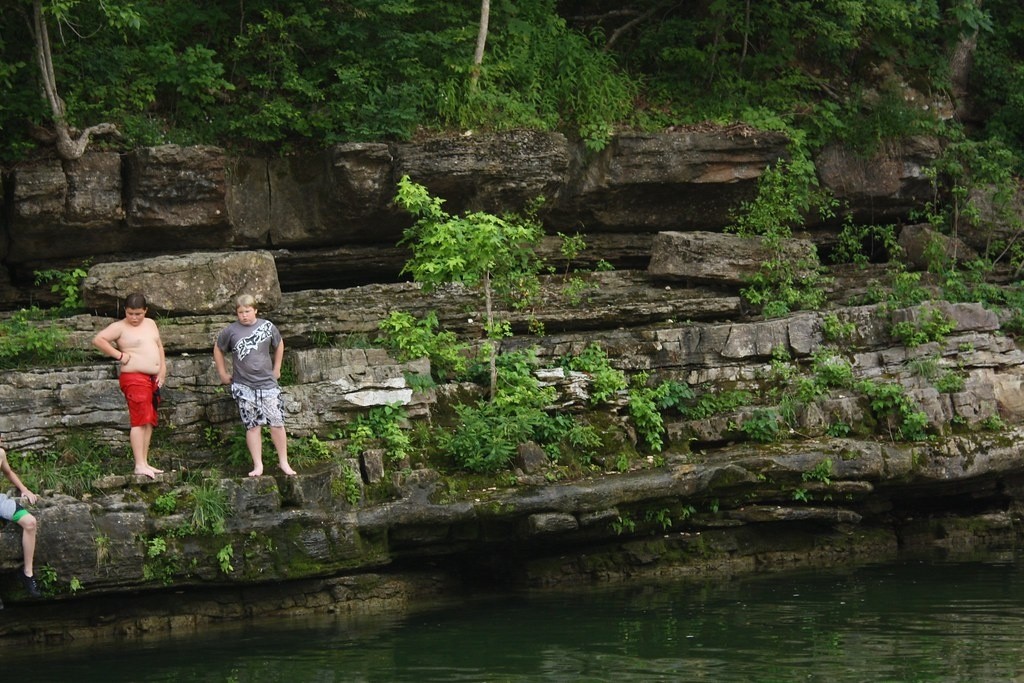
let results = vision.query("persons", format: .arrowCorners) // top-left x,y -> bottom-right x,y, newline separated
92,292 -> 169,480
210,295 -> 297,478
0,446 -> 42,598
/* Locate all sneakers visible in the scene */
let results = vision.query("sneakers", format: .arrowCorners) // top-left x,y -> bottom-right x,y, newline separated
20,569 -> 42,597
0,598 -> 5,610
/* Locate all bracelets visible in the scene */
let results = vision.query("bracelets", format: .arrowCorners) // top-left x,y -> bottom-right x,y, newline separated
118,351 -> 123,361
